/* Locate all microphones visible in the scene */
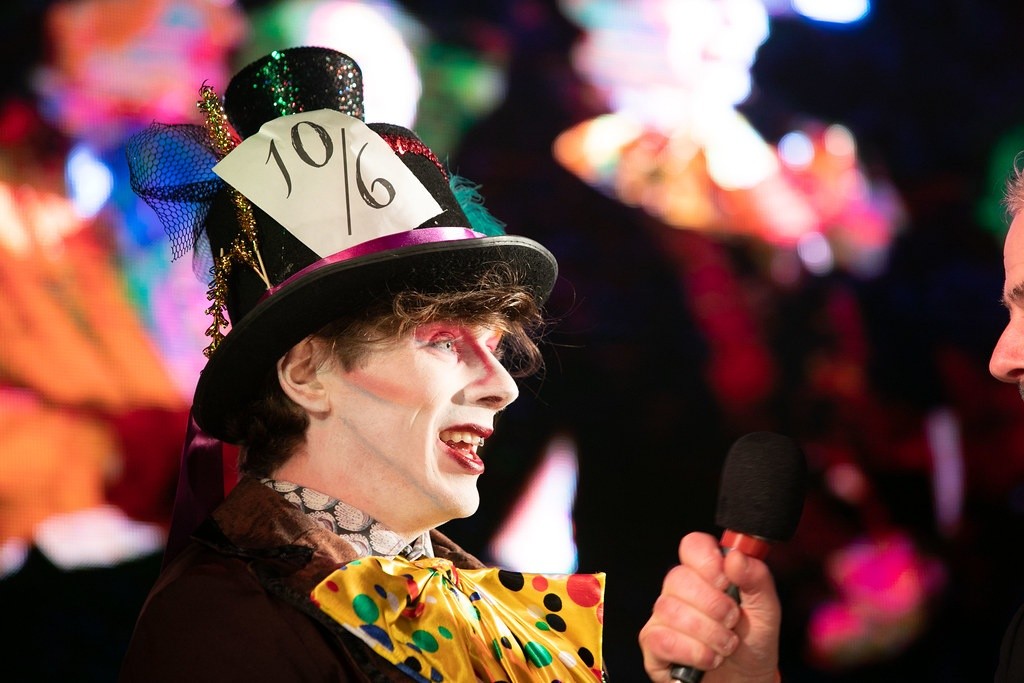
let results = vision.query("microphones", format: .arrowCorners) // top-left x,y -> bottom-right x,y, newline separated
668,431 -> 805,683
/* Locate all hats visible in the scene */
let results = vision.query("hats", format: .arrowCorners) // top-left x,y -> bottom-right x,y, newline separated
181,44 -> 561,441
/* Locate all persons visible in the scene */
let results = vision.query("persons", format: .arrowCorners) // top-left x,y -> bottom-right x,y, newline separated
125,46 -> 606,682
442,0 -> 1023,572
0,0 -> 249,682
639,150 -> 1024,683
